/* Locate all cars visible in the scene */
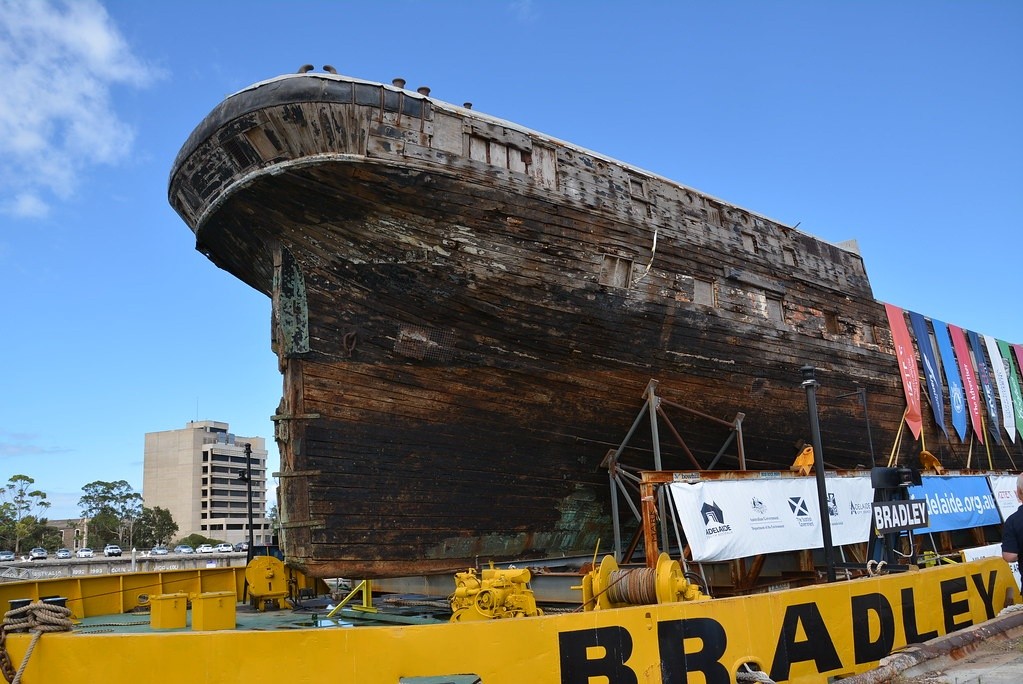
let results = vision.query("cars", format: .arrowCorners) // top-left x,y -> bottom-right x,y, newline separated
103,544 -> 122,556
76,547 -> 93,558
28,547 -> 47,559
0,550 -> 17,562
56,548 -> 72,559
234,542 -> 248,552
195,544 -> 212,554
216,542 -> 233,552
152,546 -> 168,554
174,544 -> 192,554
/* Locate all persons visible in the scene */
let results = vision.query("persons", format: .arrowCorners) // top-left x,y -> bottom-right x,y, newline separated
1000,471 -> 1023,607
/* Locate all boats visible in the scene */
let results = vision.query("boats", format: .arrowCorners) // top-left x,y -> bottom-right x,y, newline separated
0,68 -> 1023,684
